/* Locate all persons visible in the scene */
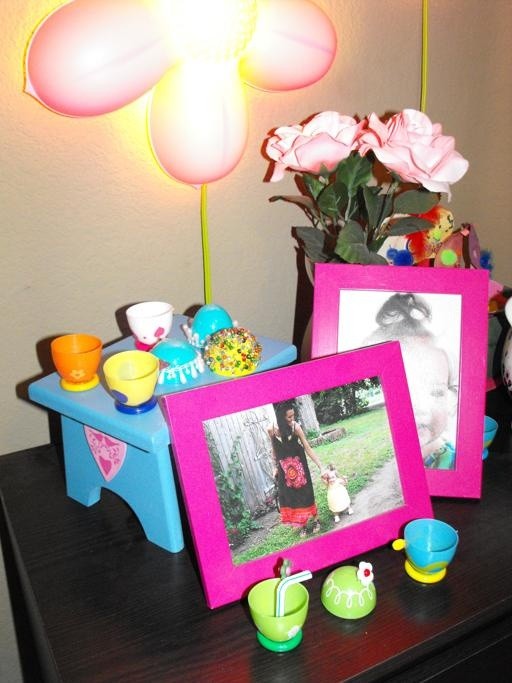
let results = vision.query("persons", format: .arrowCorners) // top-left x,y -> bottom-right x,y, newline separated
324,464 -> 354,523
361,293 -> 456,469
268,405 -> 328,537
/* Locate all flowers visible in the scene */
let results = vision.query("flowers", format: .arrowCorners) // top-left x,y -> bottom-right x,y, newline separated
261,105 -> 469,293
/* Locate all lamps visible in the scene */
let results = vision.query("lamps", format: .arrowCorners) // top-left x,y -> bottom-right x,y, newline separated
22,0 -> 338,305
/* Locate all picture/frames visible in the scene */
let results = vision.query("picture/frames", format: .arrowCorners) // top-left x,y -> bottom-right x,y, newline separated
313,263 -> 488,503
158,341 -> 435,610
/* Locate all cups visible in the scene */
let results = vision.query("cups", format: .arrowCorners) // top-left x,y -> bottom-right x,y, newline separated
392,518 -> 459,585
50,333 -> 102,392
482,415 -> 498,460
247,577 -> 310,653
126,301 -> 175,351
103,350 -> 159,415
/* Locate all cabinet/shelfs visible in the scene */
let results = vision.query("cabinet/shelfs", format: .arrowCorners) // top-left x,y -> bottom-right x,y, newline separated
0,438 -> 511,680
